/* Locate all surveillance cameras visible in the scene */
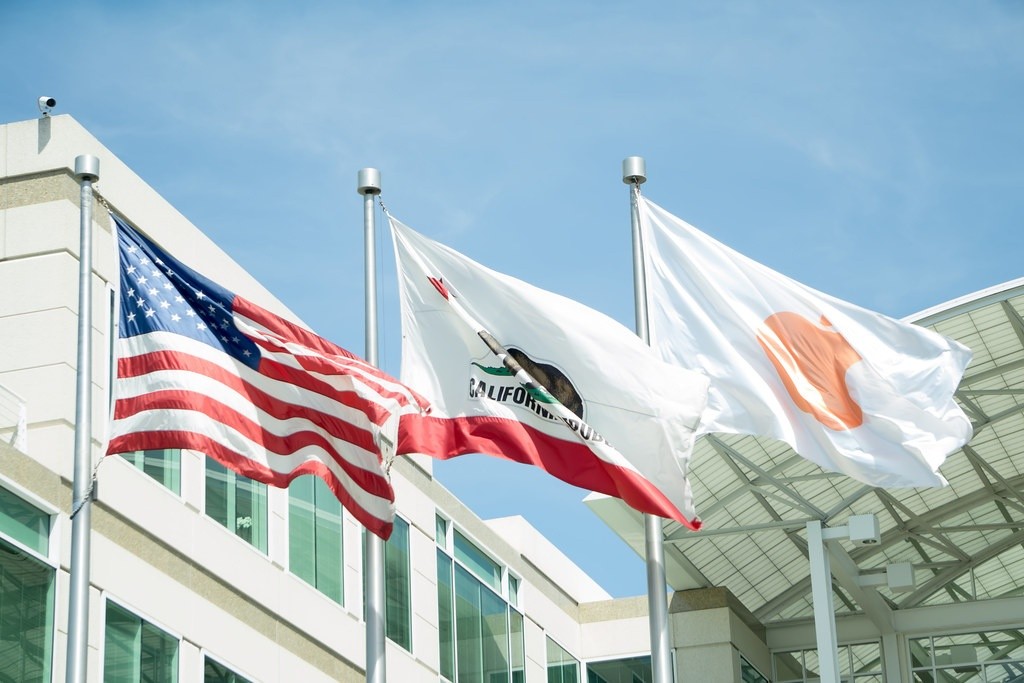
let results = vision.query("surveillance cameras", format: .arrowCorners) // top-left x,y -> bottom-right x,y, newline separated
39,96 -> 57,108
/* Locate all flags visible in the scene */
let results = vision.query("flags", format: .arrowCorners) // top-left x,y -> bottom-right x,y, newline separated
630,188 -> 975,490
70,195 -> 432,542
363,208 -> 712,532
